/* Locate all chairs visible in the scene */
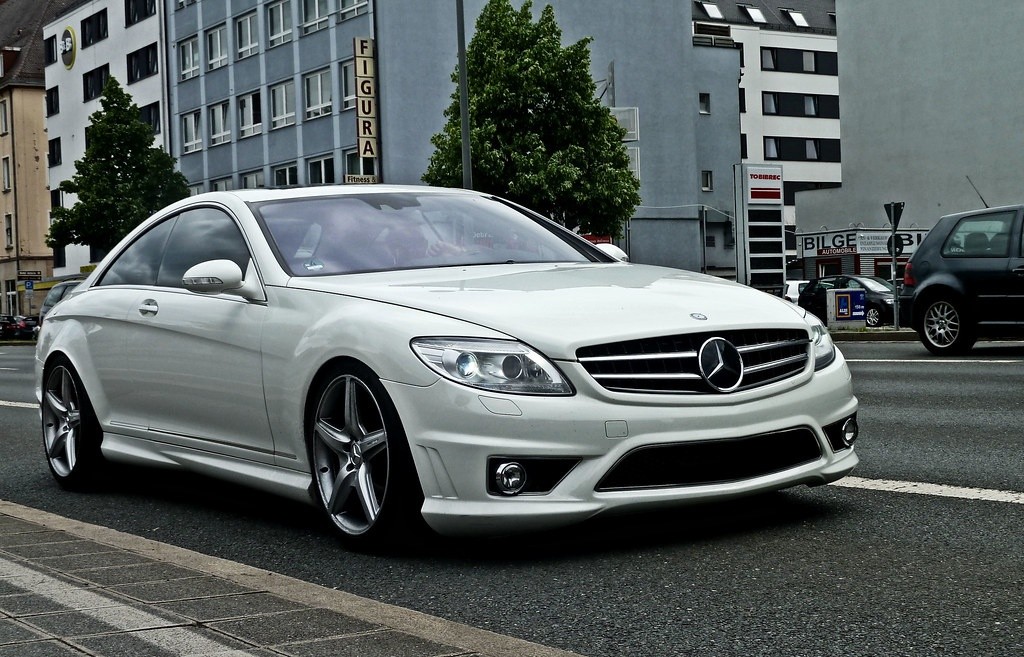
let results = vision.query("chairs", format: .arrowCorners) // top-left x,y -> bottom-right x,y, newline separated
964,232 -> 990,253
990,232 -> 1010,253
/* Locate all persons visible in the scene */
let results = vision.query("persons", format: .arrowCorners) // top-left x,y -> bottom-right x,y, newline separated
378,210 -> 466,267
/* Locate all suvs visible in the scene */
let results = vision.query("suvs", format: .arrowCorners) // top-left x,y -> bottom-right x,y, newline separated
38,276 -> 88,328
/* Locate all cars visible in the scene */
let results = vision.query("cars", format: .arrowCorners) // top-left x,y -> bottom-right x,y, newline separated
898,205 -> 1024,357
35,184 -> 861,553
798,274 -> 900,327
786,280 -> 810,306
1,315 -> 39,341
